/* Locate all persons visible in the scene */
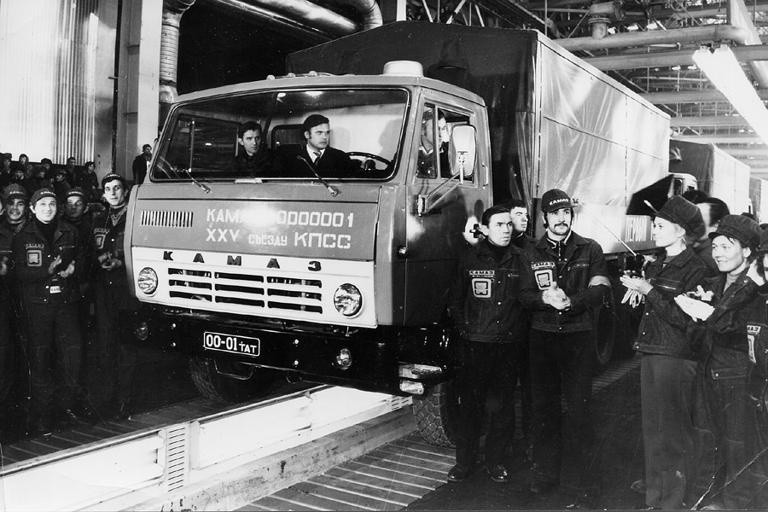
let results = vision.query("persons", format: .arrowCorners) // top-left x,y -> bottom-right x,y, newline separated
1,153 -> 144,440
133,144 -> 152,183
447,189 -> 767,511
280,113 -> 360,178
223,120 -> 285,178
383,107 -> 454,178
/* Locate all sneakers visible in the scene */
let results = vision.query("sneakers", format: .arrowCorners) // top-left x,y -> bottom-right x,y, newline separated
444,460 -> 602,510
0,391 -> 136,444
630,477 -> 748,511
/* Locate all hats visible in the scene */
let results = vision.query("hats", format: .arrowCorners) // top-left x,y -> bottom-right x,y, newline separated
708,216 -> 768,255
102,172 -> 121,179
64,188 -> 89,202
656,196 -> 704,235
542,189 -> 579,213
1,182 -> 31,205
29,187 -> 58,205
